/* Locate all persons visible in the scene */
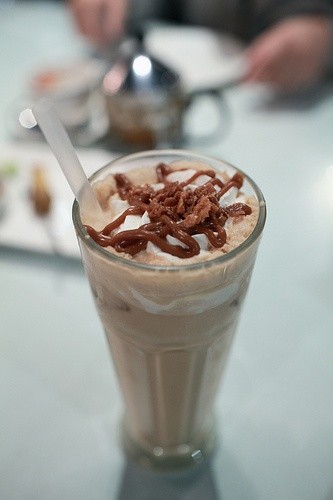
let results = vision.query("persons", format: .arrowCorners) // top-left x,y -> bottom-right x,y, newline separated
66,0 -> 333,104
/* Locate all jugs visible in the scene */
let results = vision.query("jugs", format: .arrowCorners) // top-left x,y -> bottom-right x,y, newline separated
108,22 -> 232,151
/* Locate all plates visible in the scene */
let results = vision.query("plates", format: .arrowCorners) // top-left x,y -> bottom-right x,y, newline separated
132,22 -> 255,96
1,142 -> 127,263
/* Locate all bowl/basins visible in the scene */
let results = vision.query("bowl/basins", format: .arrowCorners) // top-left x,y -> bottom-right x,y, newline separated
24,56 -> 104,127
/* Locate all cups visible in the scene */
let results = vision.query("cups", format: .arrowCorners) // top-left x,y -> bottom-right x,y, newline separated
73,149 -> 269,474
